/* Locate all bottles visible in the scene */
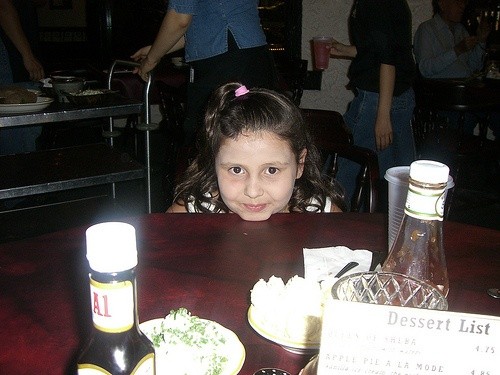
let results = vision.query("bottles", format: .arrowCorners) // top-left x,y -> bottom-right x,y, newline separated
375,160 -> 450,310
76,222 -> 157,375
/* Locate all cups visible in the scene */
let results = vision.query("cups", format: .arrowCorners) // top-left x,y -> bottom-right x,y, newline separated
331,270 -> 449,312
312,36 -> 332,69
383,166 -> 454,253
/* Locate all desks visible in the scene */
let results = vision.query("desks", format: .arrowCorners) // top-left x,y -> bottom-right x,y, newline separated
91,66 -> 187,129
0,212 -> 500,375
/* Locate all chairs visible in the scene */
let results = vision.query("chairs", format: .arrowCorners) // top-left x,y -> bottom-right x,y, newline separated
270,42 -> 309,106
300,107 -> 379,214
416,77 -> 500,229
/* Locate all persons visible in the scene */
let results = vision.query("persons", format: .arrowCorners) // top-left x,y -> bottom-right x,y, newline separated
131,0 -> 279,120
325,0 -> 416,213
0,0 -> 44,83
414,0 -> 491,77
167,81 -> 351,221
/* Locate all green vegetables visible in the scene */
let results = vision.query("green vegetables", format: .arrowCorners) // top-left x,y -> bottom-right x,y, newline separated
152,307 -> 228,375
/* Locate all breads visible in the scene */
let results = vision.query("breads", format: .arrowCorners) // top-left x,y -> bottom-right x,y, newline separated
0,87 -> 37,104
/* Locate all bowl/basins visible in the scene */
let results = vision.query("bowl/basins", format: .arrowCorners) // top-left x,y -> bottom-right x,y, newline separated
49,78 -> 85,103
65,88 -> 113,108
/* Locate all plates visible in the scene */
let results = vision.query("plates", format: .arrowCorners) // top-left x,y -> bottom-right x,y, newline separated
140,317 -> 246,375
247,303 -> 321,354
171,56 -> 188,67
0,88 -> 55,114
